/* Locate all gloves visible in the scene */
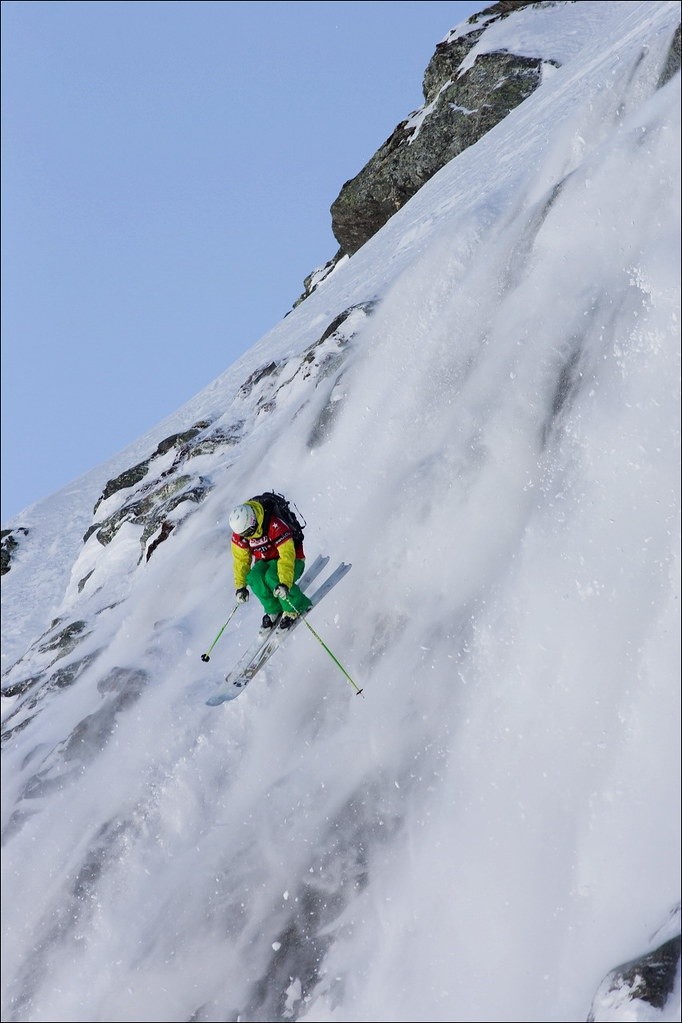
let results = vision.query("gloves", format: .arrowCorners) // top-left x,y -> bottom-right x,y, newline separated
273,585 -> 287,601
236,588 -> 249,605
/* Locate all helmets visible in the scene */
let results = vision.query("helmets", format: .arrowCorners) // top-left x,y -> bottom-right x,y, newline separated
229,503 -> 256,535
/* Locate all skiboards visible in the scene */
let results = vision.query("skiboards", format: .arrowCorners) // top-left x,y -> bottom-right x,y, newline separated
202,552 -> 353,707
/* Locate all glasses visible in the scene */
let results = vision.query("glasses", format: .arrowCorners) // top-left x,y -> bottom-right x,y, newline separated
240,527 -> 254,538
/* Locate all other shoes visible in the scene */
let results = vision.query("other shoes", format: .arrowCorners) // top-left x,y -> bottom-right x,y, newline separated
280,610 -> 296,629
263,612 -> 281,628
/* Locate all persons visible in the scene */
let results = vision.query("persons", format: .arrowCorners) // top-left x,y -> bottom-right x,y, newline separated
230,501 -> 306,629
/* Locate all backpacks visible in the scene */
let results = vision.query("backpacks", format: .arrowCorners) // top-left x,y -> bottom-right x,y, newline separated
248,490 -> 306,549
